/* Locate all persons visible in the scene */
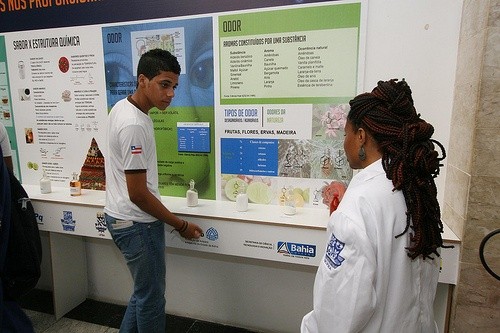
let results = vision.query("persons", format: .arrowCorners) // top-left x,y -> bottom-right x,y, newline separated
300,76 -> 446,333
105,48 -> 205,333
0,142 -> 41,332
0,118 -> 14,175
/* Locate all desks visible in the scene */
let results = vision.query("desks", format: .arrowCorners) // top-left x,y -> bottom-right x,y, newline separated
20,184 -> 461,333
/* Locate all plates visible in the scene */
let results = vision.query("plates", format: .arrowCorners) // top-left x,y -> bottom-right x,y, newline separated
245,182 -> 275,204
224,178 -> 249,201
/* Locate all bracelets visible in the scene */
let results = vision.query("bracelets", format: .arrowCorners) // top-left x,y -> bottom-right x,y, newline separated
169,218 -> 188,233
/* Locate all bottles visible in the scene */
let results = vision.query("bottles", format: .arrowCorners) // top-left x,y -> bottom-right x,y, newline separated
284,194 -> 296,216
39,173 -> 50,194
234,188 -> 248,212
69,172 -> 81,196
186,180 -> 198,207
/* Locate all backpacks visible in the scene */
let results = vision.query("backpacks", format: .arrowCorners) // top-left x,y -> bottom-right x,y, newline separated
1,144 -> 43,300
